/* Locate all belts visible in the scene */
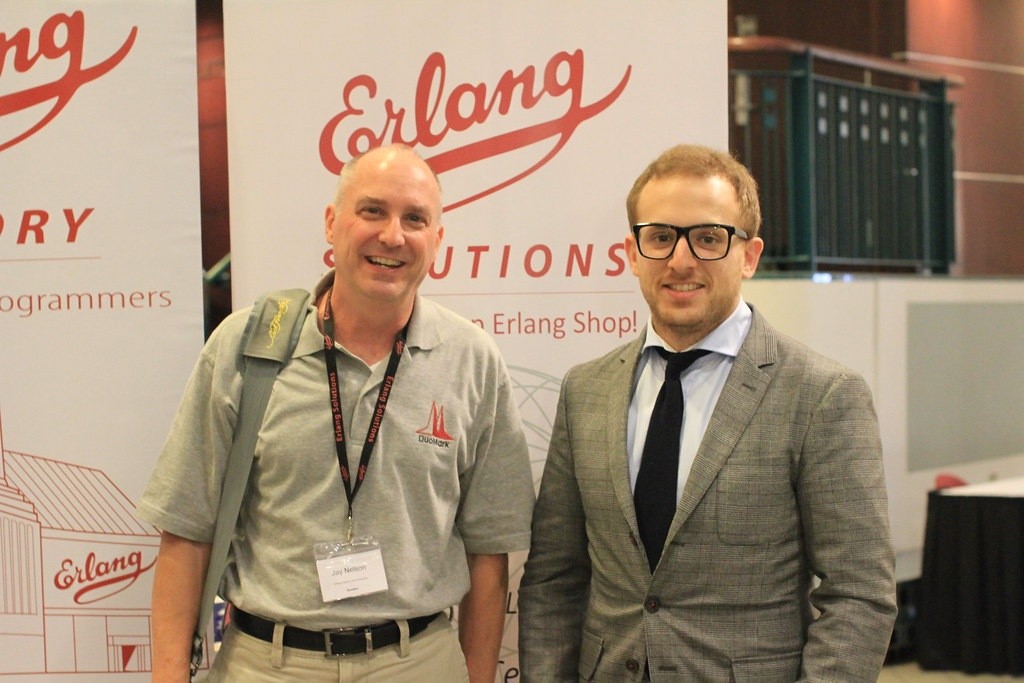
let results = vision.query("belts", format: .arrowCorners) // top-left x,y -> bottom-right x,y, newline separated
229,607 -> 444,657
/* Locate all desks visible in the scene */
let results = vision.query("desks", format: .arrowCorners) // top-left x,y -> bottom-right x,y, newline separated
915,475 -> 1024,676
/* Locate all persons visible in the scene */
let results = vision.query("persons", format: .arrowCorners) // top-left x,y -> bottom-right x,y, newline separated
132,142 -> 537,683
515,142 -> 900,683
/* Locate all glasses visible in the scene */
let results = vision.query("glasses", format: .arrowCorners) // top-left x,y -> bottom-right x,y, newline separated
632,223 -> 747,261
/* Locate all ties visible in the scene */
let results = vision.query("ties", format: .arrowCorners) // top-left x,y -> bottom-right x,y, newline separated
633,346 -> 714,574
